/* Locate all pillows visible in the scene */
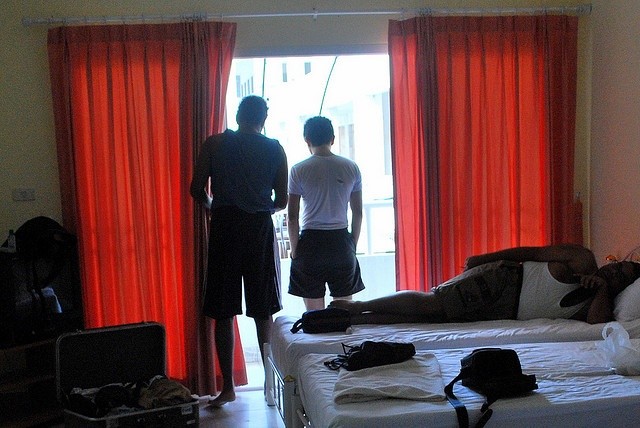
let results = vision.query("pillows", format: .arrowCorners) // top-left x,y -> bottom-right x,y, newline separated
613,279 -> 640,322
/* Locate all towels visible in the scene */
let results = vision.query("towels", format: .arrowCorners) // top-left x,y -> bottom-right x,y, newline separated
332,352 -> 446,405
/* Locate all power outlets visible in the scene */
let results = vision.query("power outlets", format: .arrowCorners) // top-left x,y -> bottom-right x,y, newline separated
12,189 -> 36,202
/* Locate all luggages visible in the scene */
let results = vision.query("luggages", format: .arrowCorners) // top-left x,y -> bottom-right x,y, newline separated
55,322 -> 199,428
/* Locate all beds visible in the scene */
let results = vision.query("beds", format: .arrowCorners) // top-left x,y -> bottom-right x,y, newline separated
262,317 -> 639,427
293,338 -> 640,428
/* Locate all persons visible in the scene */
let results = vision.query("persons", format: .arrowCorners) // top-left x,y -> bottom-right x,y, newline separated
189,95 -> 290,404
325,245 -> 640,326
286,117 -> 361,313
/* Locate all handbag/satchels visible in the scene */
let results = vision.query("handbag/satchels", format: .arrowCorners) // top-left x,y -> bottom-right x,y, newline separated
444,348 -> 538,428
290,308 -> 352,334
